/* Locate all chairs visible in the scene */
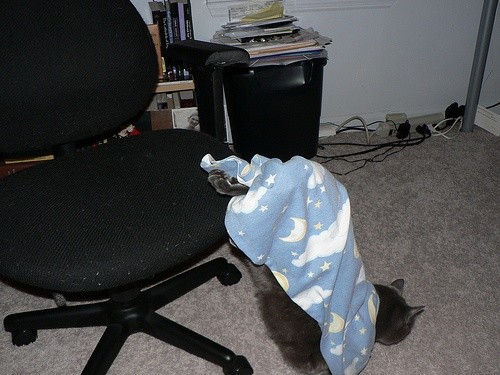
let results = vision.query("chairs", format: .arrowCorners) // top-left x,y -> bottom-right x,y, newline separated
0,0 -> 254,375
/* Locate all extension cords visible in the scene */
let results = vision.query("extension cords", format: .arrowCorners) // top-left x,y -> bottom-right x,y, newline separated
376,113 -> 444,137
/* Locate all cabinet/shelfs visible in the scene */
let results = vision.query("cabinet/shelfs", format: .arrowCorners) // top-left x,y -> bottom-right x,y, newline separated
0,80 -> 199,177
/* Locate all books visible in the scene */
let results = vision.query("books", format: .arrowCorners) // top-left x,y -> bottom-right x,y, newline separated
154,92 -> 181,110
144,0 -> 194,83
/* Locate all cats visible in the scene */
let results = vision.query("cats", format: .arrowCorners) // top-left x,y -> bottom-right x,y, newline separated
208,168 -> 427,374
